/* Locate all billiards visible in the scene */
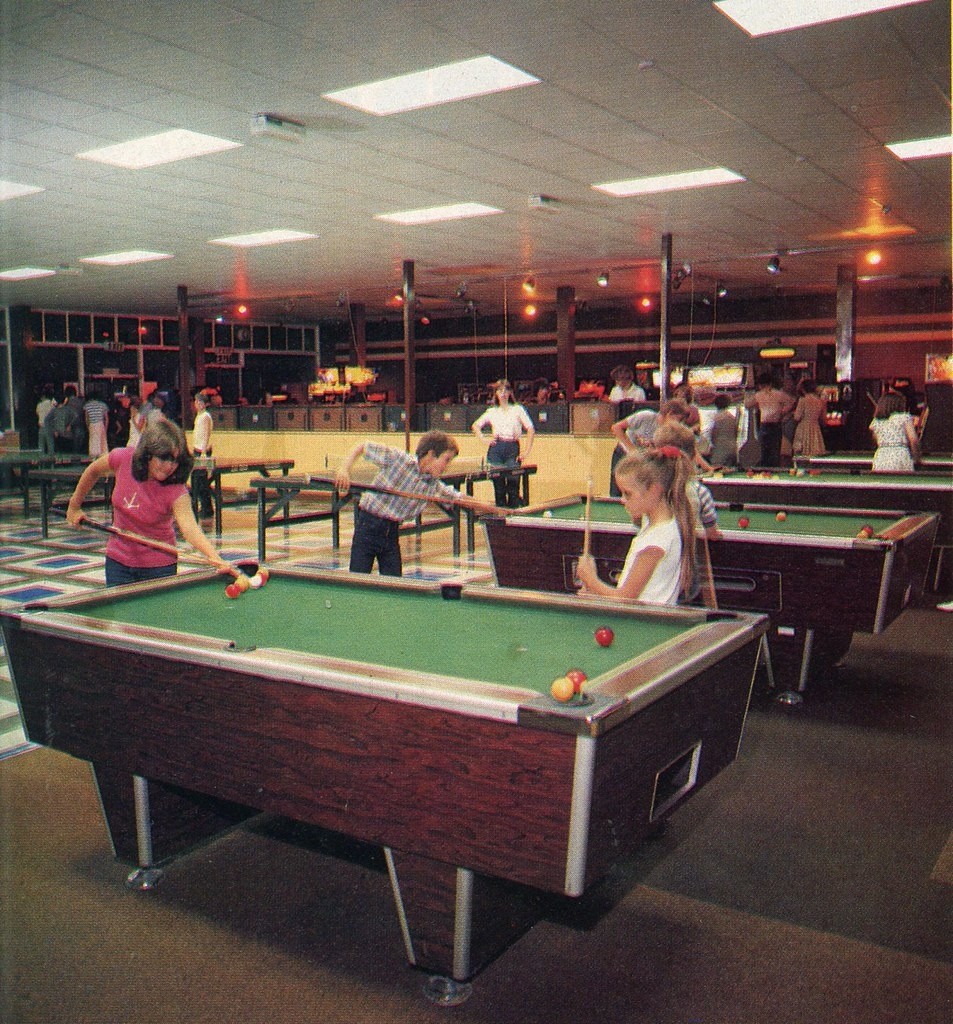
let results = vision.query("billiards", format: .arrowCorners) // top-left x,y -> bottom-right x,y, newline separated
565,670 -> 588,693
777,512 -> 787,521
225,566 -> 270,599
737,516 -> 749,527
543,510 -> 553,518
858,524 -> 873,538
595,626 -> 614,647
552,677 -> 575,702
747,468 -> 822,480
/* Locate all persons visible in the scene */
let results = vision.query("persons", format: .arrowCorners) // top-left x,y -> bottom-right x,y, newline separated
575,446 -> 699,605
191,393 -> 214,523
471,379 -> 535,508
608,364 -> 827,497
66,418 -> 242,588
652,420 -> 724,604
334,429 -> 476,578
35,384 -> 179,490
868,391 -> 922,473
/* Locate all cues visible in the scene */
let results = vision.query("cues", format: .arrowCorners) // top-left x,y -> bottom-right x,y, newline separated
580,480 -> 593,592
305,476 -> 516,514
867,392 -> 878,407
702,528 -> 719,610
48,507 -> 236,569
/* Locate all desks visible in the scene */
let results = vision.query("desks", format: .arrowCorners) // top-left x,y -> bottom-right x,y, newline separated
0,561 -> 771,1009
0,449 -> 952,708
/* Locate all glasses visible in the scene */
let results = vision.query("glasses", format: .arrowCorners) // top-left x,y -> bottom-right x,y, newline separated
157,453 -> 180,464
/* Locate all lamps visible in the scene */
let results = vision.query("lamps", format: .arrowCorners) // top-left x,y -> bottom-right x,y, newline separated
252,116 -> 893,325
